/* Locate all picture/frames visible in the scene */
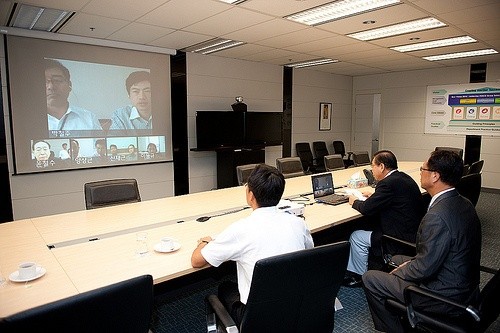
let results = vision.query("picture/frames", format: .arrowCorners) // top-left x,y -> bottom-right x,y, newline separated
319,102 -> 332,130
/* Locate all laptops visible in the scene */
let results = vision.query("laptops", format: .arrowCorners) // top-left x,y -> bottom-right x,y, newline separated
311,173 -> 349,205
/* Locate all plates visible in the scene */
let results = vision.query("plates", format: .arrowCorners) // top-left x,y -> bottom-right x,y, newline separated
8,267 -> 47,283
156,243 -> 181,254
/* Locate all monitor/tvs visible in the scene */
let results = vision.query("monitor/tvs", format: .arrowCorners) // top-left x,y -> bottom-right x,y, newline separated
195,110 -> 283,150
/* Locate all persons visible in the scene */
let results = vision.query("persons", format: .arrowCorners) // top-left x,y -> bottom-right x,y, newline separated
31,59 -> 158,167
343,150 -> 423,287
362,150 -> 482,333
192,164 -> 314,332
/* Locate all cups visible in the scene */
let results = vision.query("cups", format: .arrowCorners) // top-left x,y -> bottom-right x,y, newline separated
18,261 -> 37,276
161,238 -> 174,250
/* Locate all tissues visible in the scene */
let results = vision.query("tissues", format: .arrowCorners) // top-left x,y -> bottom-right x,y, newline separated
346,170 -> 368,189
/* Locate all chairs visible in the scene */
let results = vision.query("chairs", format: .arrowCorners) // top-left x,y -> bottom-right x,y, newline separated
324,154 -> 346,172
237,163 -> 264,184
461,160 -> 485,209
379,235 -> 420,270
436,147 -> 463,160
380,265 -> 500,333
276,157 -> 305,178
353,151 -> 371,166
0,274 -> 154,333
333,141 -> 353,164
84,178 -> 141,210
295,142 -> 313,171
313,142 -> 329,171
208,240 -> 351,333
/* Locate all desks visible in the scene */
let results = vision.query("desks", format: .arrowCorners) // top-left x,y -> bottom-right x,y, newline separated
0,161 -> 431,322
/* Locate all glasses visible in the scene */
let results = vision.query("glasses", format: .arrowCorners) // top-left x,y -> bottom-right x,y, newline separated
420,166 -> 435,172
46,77 -> 68,86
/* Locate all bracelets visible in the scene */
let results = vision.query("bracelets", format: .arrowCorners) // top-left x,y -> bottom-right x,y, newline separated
202,240 -> 209,244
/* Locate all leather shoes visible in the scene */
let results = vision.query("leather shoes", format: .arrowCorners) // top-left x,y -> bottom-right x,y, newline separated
342,274 -> 362,287
